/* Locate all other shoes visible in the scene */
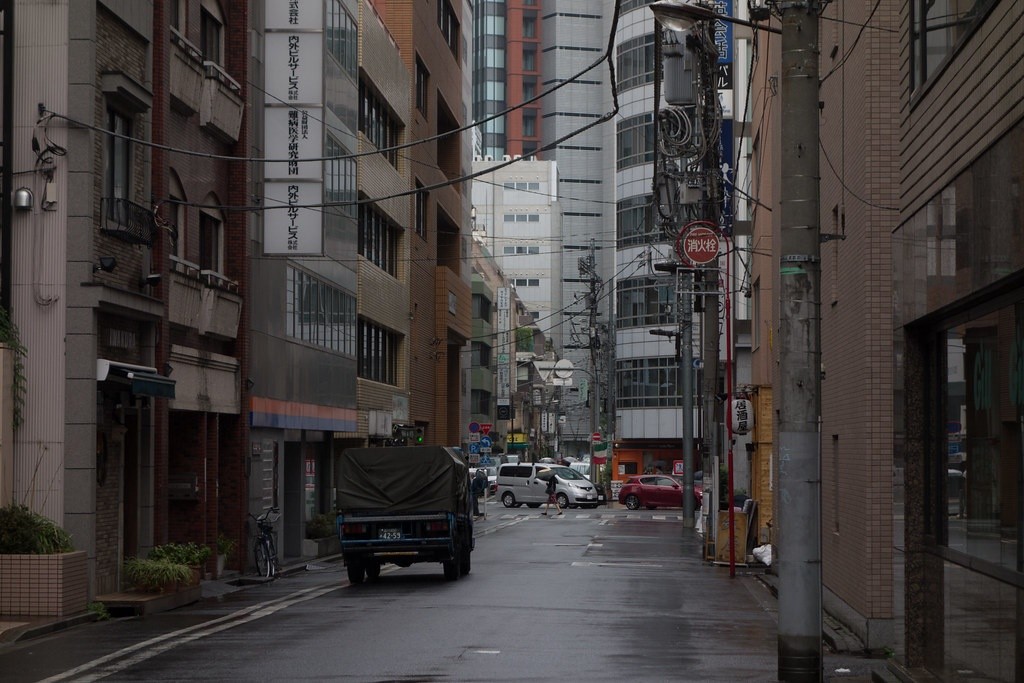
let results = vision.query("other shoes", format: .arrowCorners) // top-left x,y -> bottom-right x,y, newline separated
541,513 -> 547,515
558,512 -> 562,515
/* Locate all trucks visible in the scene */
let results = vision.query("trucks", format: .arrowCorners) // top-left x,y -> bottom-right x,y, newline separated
335,444 -> 474,586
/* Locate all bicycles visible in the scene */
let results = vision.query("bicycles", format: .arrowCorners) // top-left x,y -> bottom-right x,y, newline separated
252,506 -> 280,577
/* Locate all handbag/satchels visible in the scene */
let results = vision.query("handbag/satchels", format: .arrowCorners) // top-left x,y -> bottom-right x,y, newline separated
545,485 -> 554,494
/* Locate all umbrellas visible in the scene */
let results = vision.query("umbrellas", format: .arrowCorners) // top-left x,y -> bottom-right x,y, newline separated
537,468 -> 556,480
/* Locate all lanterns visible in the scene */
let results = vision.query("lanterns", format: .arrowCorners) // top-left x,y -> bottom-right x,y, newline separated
726,399 -> 754,435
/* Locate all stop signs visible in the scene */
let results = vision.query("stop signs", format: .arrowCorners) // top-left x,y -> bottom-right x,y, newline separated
592,433 -> 602,442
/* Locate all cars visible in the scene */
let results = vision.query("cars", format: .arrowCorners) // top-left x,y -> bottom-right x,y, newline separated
494,463 -> 598,509
618,475 -> 703,511
569,463 -> 591,480
468,465 -> 498,491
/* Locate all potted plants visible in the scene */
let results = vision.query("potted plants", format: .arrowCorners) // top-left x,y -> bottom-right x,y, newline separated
215,533 -> 233,577
304,509 -> 341,558
120,541 -> 212,595
0,505 -> 88,617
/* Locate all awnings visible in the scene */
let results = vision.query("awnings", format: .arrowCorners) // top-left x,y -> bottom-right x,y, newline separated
123,369 -> 176,398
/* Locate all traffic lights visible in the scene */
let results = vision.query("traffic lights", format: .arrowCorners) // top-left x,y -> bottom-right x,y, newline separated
415,426 -> 424,444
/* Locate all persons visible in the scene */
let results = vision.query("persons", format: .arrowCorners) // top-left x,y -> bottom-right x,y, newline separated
541,475 -> 562,515
